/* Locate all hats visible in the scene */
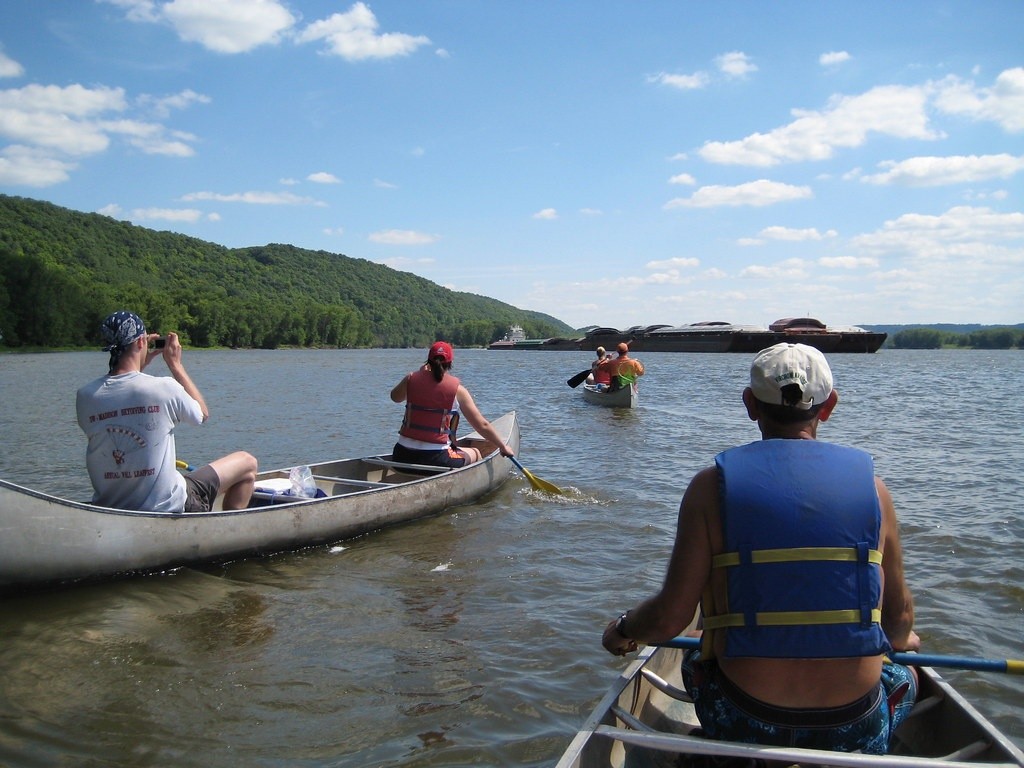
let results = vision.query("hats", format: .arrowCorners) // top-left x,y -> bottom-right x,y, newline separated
429,341 -> 453,363
617,343 -> 628,352
101,311 -> 146,351
750,342 -> 833,410
597,346 -> 605,356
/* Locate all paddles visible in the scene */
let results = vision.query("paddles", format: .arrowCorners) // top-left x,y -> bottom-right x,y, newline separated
508,456 -> 563,494
176,460 -> 198,472
567,336 -> 636,389
652,635 -> 1024,675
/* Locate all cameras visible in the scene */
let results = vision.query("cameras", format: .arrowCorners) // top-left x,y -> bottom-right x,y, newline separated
154,338 -> 165,349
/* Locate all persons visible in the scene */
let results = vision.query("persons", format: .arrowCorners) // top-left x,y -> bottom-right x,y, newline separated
390,342 -> 514,476
585,342 -> 644,392
602,342 -> 921,756
76,311 -> 257,513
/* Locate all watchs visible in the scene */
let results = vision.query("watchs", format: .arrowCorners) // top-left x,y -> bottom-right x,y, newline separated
615,610 -> 632,639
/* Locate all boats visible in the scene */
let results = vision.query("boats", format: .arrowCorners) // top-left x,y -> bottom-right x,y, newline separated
582,377 -> 638,411
574,317 -> 888,352
0,409 -> 522,584
